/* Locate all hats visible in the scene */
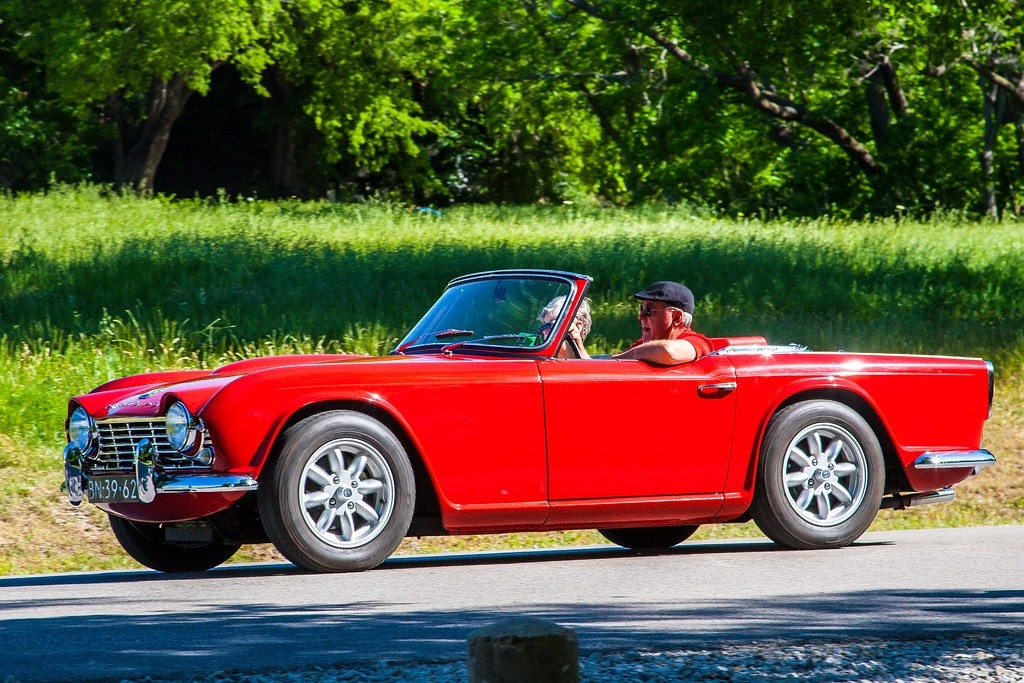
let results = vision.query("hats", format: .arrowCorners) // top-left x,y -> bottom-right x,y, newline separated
633,281 -> 694,315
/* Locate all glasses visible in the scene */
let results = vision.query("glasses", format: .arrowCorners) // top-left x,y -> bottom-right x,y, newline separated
638,304 -> 676,320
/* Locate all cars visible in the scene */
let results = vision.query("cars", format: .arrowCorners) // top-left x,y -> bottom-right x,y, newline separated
60,268 -> 996,573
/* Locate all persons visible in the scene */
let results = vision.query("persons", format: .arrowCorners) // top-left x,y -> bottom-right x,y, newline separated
536,295 -> 592,360
567,281 -> 714,365
